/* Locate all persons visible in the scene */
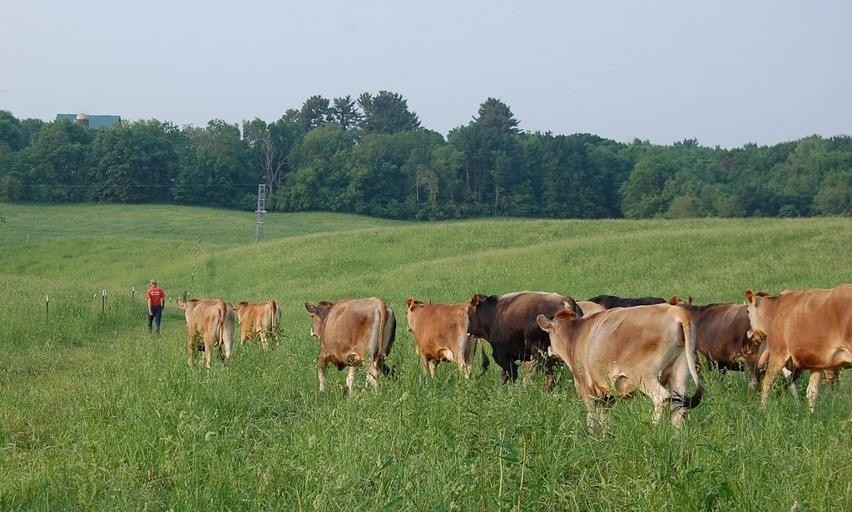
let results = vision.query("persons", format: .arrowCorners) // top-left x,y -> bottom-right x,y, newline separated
145,277 -> 167,336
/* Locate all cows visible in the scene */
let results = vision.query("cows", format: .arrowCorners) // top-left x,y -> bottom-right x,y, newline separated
404,294 -> 490,382
177,297 -> 235,374
465,289 -> 585,394
302,296 -> 397,398
231,300 -> 282,354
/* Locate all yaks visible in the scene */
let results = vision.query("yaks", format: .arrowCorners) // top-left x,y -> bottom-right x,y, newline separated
670,295 -> 765,396
744,282 -> 852,413
587,293 -> 666,308
536,302 -> 705,430
